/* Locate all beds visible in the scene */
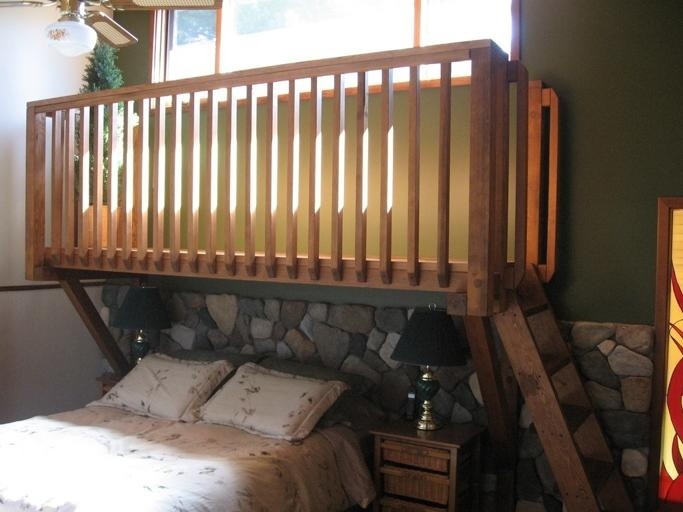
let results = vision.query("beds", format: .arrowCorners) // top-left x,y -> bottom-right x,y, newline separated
0,393 -> 385,512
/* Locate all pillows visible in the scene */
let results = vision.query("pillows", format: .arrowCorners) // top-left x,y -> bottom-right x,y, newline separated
84,347 -> 377,442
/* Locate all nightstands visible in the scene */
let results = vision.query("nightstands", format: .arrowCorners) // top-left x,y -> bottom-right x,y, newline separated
98,374 -> 128,395
367,415 -> 485,512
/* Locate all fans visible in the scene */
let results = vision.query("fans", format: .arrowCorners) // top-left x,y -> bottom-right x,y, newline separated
0,0 -> 222,47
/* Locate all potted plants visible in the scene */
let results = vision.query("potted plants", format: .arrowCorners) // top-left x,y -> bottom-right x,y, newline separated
73,42 -> 141,249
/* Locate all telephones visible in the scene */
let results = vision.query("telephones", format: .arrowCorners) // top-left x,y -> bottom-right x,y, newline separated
405,385 -> 417,421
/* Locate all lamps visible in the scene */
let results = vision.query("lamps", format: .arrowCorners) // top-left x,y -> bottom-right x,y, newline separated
108,284 -> 174,368
42,12 -> 97,59
388,300 -> 469,432
